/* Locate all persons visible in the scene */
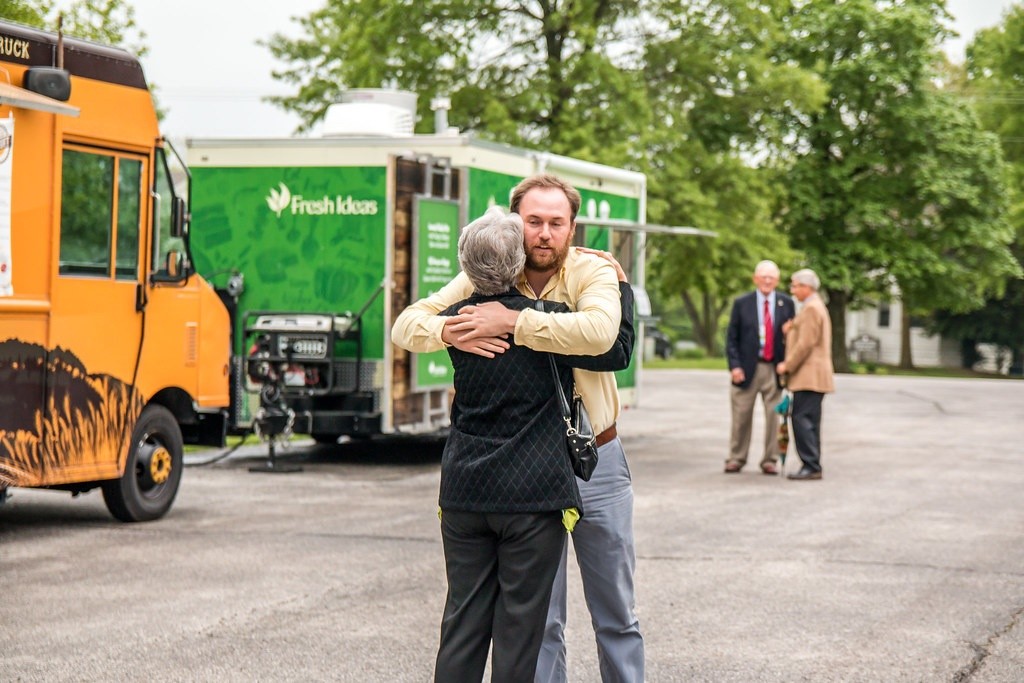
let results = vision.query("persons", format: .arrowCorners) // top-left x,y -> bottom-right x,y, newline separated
724,260 -> 795,474
390,171 -> 645,683
434,204 -> 623,683
776,268 -> 836,479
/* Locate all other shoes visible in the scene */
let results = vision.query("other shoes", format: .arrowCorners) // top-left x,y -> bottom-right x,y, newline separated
725,461 -> 740,472
788,465 -> 822,480
763,464 -> 779,475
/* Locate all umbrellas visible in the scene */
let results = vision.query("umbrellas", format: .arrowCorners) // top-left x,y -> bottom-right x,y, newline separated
773,372 -> 793,476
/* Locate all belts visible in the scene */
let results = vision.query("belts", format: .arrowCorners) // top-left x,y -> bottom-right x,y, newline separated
593,423 -> 618,446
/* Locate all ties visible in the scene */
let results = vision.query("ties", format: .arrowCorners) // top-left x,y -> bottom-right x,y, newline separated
762,299 -> 774,362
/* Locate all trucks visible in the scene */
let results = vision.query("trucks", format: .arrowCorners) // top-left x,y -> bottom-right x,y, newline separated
178,87 -> 722,455
0,15 -> 243,525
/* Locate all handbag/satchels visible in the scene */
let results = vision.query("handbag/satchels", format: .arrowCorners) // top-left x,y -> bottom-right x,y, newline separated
567,394 -> 598,482
